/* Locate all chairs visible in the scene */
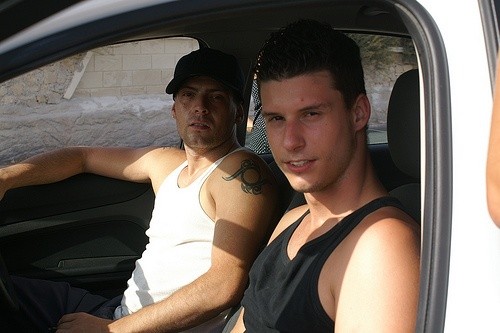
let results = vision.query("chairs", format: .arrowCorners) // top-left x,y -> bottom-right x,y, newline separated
368,66 -> 421,224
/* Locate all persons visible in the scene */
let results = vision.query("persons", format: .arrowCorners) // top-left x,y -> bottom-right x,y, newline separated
216,17 -> 422,333
1,47 -> 274,333
